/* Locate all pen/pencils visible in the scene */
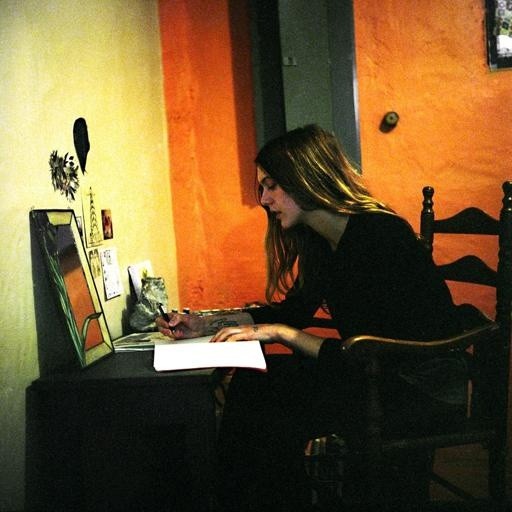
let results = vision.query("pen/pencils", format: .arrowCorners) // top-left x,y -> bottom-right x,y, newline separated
158,304 -> 177,335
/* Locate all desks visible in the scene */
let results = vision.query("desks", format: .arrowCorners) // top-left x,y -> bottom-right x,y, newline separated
31,331 -> 237,512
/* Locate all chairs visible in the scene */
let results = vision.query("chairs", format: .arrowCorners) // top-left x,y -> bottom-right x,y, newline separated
328,181 -> 512,512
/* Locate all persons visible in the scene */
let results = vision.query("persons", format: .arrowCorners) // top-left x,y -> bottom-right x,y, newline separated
157,124 -> 467,512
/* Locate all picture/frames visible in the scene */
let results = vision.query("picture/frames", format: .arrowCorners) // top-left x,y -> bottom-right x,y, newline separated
484,0 -> 512,71
28,209 -> 115,367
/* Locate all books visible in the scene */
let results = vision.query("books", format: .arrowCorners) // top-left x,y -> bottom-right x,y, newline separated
114,332 -> 156,352
154,334 -> 268,371
